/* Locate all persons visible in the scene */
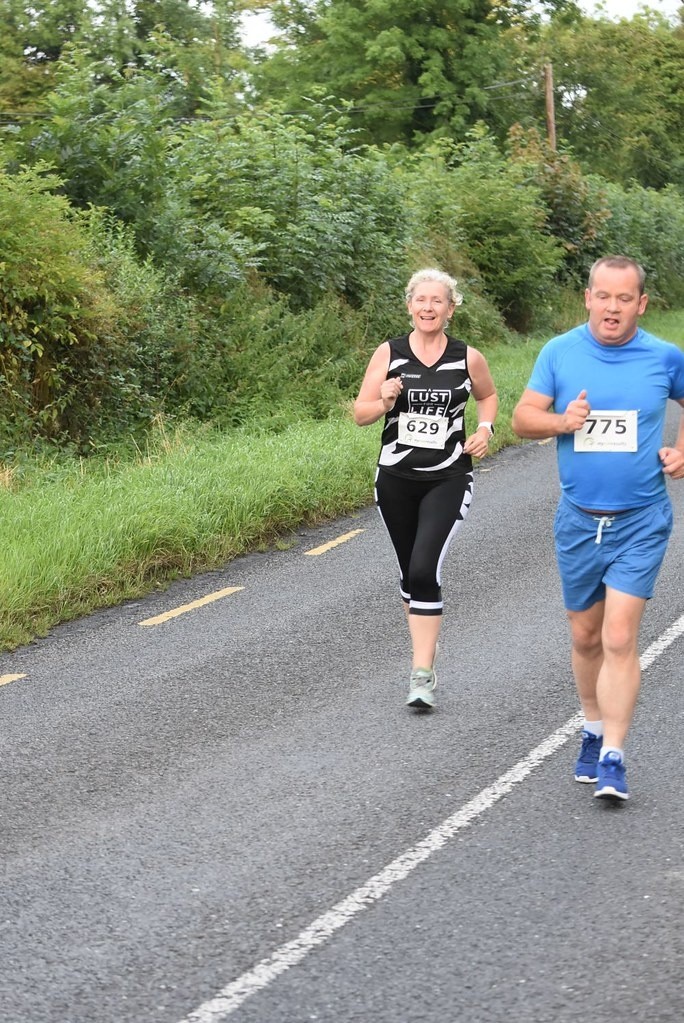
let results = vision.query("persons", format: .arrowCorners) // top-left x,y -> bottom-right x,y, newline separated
353,270 -> 498,711
509,254 -> 684,801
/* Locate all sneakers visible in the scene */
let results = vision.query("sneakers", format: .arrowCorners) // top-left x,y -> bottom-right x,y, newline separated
594,751 -> 629,802
406,667 -> 437,709
431,642 -> 439,692
573,730 -> 603,783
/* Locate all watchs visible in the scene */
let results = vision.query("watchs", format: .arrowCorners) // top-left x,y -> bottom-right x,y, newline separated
476,421 -> 496,440
